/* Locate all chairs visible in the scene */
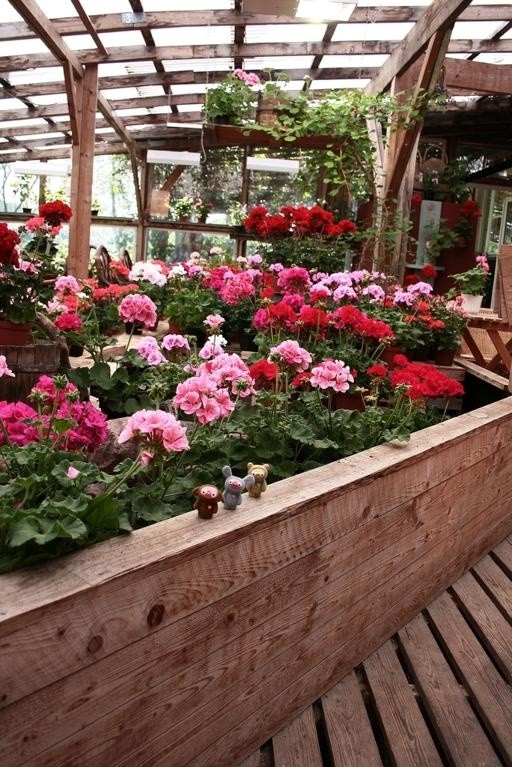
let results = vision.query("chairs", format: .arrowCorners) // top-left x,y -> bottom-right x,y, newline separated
452,244 -> 512,381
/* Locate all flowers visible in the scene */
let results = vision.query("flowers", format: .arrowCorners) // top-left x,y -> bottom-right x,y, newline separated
202,67 -> 261,124
447,254 -> 491,296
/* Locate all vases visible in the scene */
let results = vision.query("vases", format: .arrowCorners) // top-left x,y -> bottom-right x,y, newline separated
462,293 -> 483,315
214,115 -> 231,124
